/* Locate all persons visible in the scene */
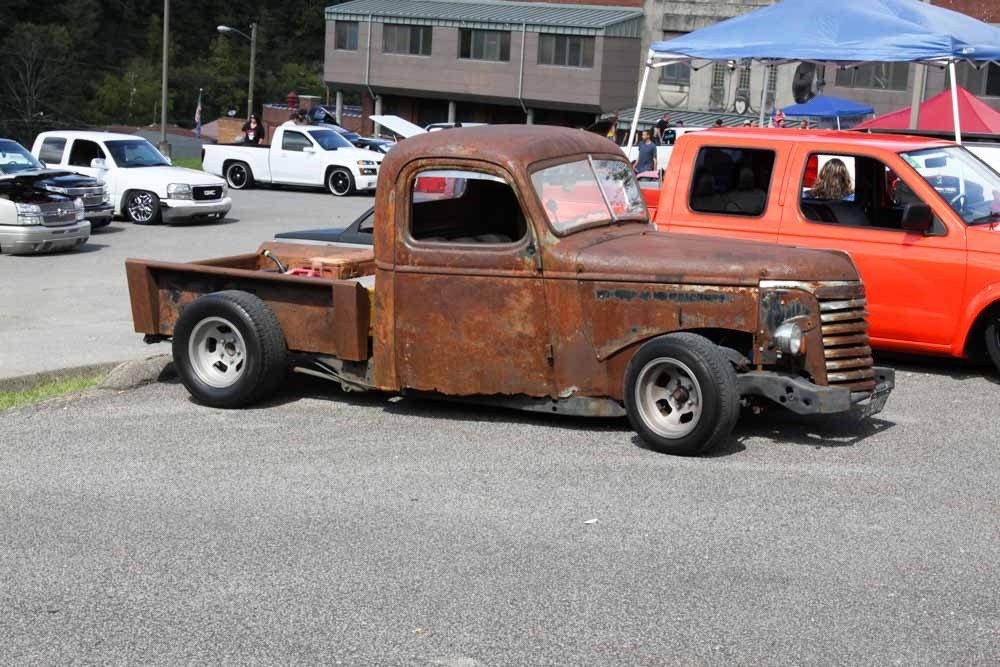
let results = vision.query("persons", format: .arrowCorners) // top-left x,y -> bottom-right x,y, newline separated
774,109 -> 785,128
656,112 -> 670,145
282,109 -> 307,126
241,113 -> 265,144
743,117 -> 759,127
633,130 -> 657,182
715,119 -> 722,126
803,158 -> 855,201
799,120 -> 808,130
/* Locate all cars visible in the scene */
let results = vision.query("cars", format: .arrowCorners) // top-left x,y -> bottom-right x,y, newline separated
0,137 -> 118,256
284,114 -> 487,154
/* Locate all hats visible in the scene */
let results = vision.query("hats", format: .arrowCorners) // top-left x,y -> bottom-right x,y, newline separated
744,119 -> 751,125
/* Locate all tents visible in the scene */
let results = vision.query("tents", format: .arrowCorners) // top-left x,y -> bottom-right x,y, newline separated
624,0 -> 1000,205
780,95 -> 875,131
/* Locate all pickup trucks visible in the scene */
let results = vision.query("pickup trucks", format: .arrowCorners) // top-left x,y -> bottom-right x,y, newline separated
406,126 -> 1000,382
124,124 -> 894,457
201,119 -> 386,198
0,130 -> 233,226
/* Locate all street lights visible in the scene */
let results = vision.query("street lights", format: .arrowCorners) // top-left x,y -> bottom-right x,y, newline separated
218,23 -> 258,121
